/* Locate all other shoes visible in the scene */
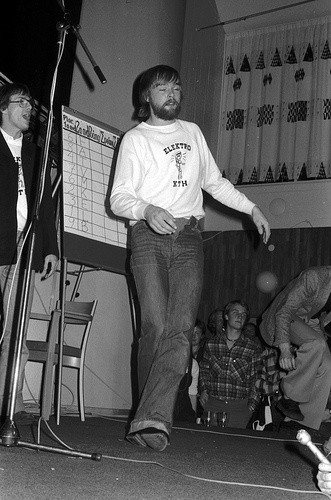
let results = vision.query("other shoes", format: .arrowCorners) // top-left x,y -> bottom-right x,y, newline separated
126,427 -> 169,452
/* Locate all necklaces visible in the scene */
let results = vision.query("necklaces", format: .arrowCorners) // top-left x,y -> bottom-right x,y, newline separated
226,334 -> 241,341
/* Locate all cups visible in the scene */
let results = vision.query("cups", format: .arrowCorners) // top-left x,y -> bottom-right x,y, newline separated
204,410 -> 213,427
219,412 -> 229,428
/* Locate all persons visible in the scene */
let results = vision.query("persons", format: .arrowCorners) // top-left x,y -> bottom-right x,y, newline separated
0,81 -> 59,426
172,305 -> 331,437
110,63 -> 271,451
259,265 -> 331,439
198,300 -> 263,429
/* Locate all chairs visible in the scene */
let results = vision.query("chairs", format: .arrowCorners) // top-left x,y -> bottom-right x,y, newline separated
24,299 -> 98,426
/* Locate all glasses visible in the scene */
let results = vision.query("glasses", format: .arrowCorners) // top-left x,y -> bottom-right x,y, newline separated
9,98 -> 35,110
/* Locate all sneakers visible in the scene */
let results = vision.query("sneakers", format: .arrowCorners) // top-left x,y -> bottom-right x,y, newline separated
276,395 -> 304,421
